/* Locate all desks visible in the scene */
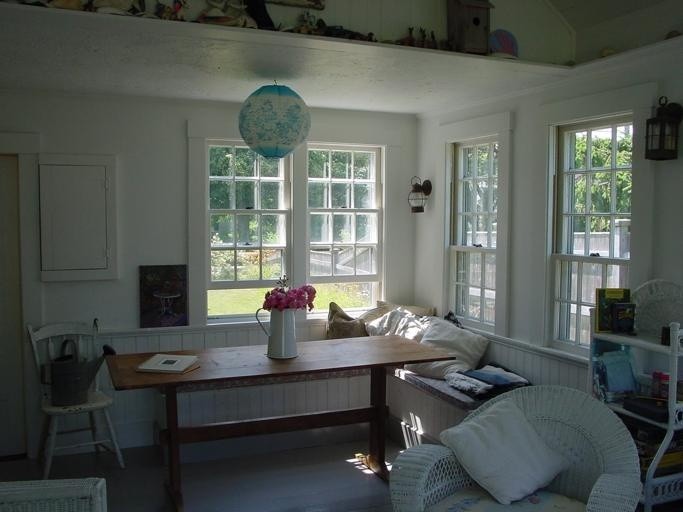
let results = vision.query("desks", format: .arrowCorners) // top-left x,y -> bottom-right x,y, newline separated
105,337 -> 454,512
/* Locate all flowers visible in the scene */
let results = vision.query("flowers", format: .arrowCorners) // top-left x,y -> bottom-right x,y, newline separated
262,285 -> 317,312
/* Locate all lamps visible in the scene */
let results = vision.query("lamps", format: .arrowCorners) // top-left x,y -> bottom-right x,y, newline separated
643,94 -> 683,161
407,171 -> 432,214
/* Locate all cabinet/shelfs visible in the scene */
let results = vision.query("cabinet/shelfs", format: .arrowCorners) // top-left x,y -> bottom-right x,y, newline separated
588,278 -> 683,512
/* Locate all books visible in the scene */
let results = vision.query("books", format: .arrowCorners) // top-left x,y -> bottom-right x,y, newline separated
632,424 -> 683,483
595,287 -> 631,332
611,302 -> 636,335
135,354 -> 199,374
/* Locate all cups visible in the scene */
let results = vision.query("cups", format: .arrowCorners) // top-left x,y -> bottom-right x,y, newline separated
255,306 -> 300,360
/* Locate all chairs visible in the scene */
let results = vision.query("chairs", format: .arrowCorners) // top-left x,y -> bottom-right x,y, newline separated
0,475 -> 108,512
27,318 -> 128,480
389,385 -> 640,512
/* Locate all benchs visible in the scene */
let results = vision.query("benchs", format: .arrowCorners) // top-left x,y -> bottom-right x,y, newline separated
386,368 -> 528,462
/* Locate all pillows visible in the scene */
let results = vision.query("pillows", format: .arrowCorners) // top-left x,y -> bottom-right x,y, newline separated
326,300 -> 490,381
444,360 -> 526,401
439,399 -> 572,504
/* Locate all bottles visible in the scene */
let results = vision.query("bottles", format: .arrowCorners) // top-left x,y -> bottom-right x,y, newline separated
651,371 -> 662,397
661,373 -> 670,400
